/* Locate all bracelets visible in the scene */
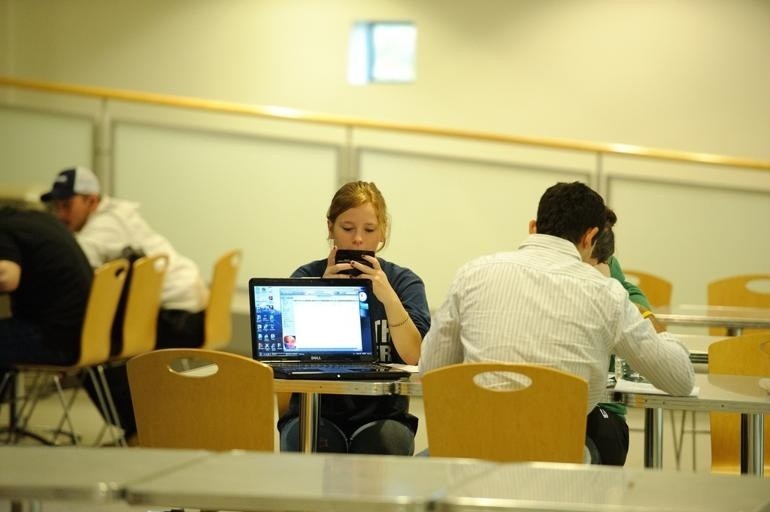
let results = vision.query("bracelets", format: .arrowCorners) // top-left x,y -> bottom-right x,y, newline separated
643,311 -> 652,318
389,317 -> 409,327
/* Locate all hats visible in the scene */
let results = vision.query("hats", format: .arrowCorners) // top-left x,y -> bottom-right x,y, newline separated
39,165 -> 101,203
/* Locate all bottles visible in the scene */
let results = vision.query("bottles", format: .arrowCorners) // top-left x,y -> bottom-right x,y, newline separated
616,357 -> 639,383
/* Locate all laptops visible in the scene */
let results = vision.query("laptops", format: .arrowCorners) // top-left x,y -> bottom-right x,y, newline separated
249,278 -> 411,380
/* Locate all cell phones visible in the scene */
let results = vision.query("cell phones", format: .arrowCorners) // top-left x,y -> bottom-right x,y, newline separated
335,250 -> 375,276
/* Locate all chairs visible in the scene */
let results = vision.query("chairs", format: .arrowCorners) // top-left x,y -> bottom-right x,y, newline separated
14,254 -> 168,447
195,248 -> 242,350
708,334 -> 770,476
707,274 -> 770,336
421,361 -> 589,464
126,348 -> 274,451
0,258 -> 130,447
623,270 -> 673,330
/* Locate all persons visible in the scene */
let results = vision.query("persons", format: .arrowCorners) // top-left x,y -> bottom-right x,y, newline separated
40,167 -> 207,445
0,194 -> 93,370
418,181 -> 694,465
275,181 -> 432,454
580,208 -> 651,418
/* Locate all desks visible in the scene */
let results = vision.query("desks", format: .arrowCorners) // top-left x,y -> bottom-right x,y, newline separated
652,303 -> 770,336
684,335 -> 729,363
274,363 -> 615,453
614,363 -> 769,477
